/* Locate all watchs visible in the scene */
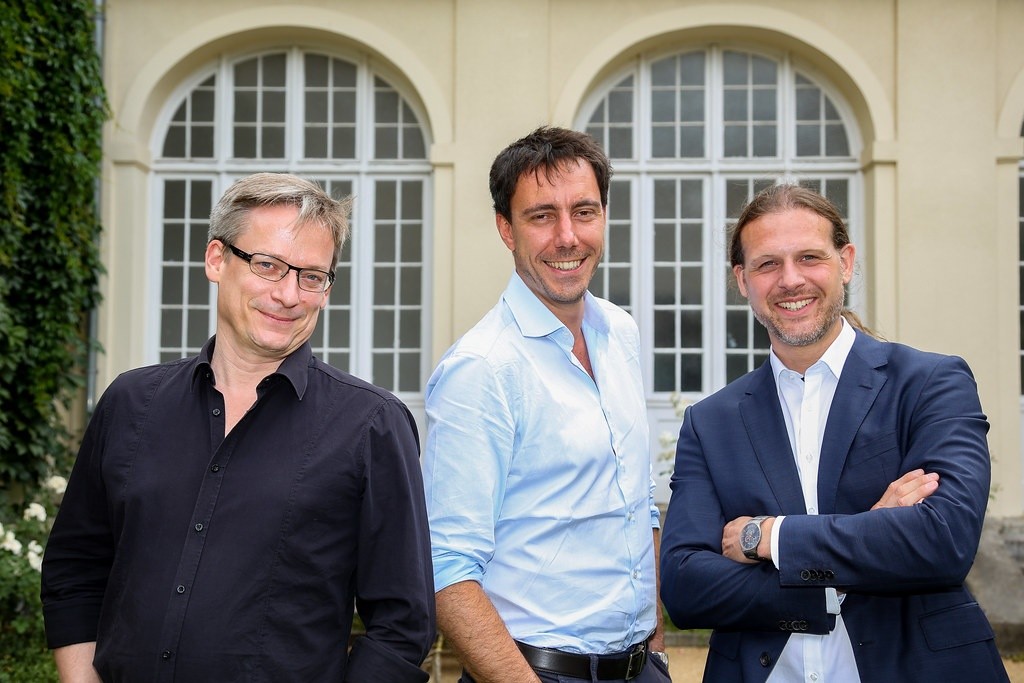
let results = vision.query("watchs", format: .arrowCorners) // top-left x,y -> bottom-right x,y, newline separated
740,515 -> 770,560
652,651 -> 668,666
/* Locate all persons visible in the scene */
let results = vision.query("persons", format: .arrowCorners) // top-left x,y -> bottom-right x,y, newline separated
421,128 -> 673,683
39,171 -> 435,683
657,182 -> 1009,683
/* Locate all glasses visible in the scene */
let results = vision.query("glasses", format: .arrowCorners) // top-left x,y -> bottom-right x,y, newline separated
216,238 -> 336,294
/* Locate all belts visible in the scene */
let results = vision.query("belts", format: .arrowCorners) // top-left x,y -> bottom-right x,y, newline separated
512,639 -> 647,682
